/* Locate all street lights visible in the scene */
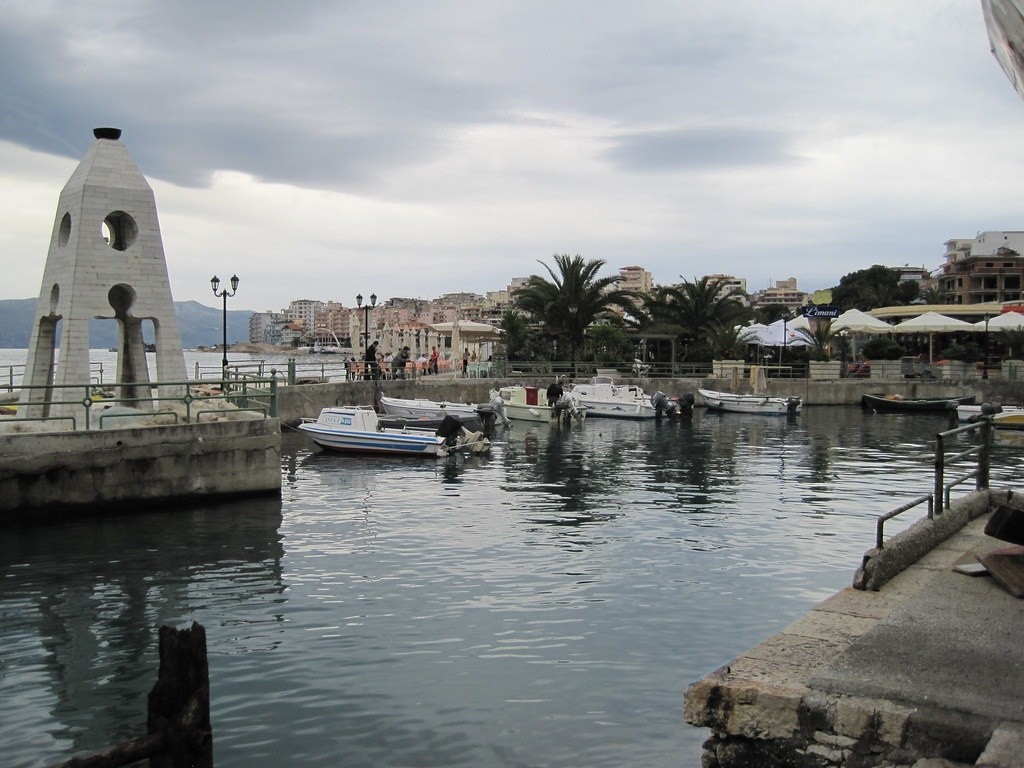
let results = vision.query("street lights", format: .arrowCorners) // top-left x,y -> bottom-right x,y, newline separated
983,312 -> 990,380
211,274 -> 239,391
357,293 -> 377,379
782,308 -> 790,360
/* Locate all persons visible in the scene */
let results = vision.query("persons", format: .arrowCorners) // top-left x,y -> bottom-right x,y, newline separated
486,356 -> 492,362
418,354 -> 427,375
428,355 -> 433,374
343,354 -> 393,381
432,346 -> 439,374
461,348 -> 470,377
391,346 -> 410,380
367,341 -> 382,379
547,381 -> 563,407
472,350 -> 476,360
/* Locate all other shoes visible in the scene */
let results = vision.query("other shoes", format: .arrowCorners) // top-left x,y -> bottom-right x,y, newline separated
402,377 -> 406,379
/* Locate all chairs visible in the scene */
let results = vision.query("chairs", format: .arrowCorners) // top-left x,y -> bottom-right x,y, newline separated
350,360 -> 491,378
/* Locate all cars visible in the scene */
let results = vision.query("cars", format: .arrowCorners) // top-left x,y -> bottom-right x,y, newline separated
832,353 -> 871,377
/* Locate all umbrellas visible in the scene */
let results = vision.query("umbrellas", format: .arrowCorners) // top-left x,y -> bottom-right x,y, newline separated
734,314 -> 810,373
831,308 -> 893,364
892,311 -> 975,362
972,311 -> 1024,355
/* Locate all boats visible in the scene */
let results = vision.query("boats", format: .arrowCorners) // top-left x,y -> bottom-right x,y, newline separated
298,405 -> 450,458
862,393 -> 976,419
380,396 -> 478,421
489,389 -> 596,423
698,389 -> 802,412
956,405 -> 1024,424
569,377 -> 668,417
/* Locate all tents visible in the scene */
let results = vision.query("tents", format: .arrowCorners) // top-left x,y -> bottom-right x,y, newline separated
428,320 -> 504,361
